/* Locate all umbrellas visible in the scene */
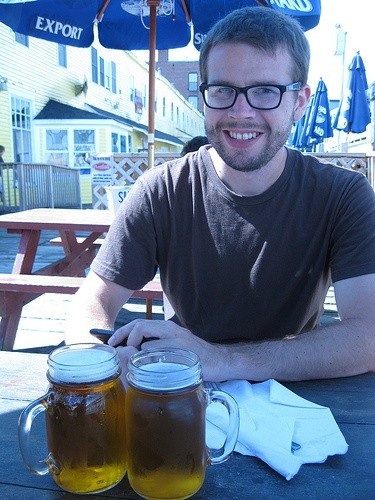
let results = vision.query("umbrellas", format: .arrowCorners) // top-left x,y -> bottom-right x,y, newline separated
287,76 -> 333,154
332,51 -> 372,153
0,0 -> 323,175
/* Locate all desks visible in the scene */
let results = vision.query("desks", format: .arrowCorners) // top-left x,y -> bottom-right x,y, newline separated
0,351 -> 375,500
1,208 -> 117,351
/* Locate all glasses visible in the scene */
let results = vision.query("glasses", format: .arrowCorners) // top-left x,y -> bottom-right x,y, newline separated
199,81 -> 301,110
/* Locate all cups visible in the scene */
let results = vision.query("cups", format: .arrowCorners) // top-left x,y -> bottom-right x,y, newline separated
124,346 -> 238,500
18,339 -> 129,494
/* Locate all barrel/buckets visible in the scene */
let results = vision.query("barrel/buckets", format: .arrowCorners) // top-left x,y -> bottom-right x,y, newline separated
104,184 -> 134,215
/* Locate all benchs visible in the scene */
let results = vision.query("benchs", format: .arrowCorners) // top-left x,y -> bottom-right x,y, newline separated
50,236 -> 104,248
0,274 -> 162,299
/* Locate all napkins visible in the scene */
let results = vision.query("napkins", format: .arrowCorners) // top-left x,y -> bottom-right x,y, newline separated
204,378 -> 349,480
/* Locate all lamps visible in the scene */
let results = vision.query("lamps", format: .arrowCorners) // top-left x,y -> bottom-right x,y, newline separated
0,74 -> 8,91
75,82 -> 87,97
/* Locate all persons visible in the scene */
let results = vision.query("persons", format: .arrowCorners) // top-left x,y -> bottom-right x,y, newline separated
180,136 -> 209,158
63,7 -> 375,394
0,145 -> 8,212
